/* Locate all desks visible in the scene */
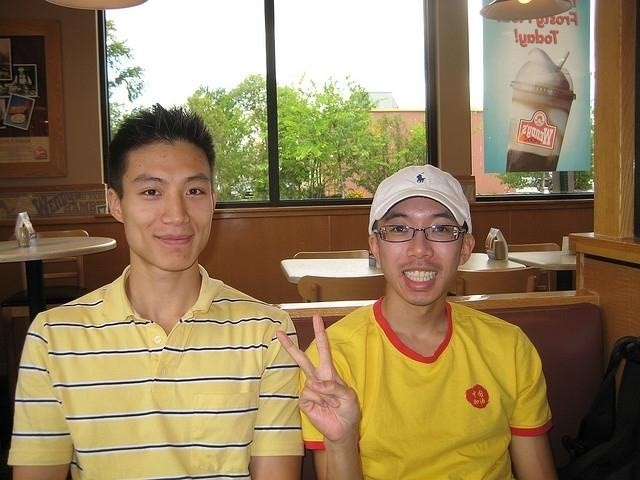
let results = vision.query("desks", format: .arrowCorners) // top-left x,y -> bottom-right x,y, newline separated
0,237 -> 118,324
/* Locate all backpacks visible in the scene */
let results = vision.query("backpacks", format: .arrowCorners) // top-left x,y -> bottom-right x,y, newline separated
556,336 -> 640,480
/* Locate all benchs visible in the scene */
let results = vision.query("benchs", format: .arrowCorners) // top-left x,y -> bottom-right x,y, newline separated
253,287 -> 604,480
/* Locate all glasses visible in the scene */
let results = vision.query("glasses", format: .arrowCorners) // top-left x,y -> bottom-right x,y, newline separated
373,225 -> 468,243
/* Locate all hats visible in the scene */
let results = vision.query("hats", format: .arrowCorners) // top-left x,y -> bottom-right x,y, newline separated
368,164 -> 473,236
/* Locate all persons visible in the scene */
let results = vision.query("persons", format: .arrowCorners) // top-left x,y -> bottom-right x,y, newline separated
6,101 -> 306,479
9,67 -> 34,88
275,162 -> 563,480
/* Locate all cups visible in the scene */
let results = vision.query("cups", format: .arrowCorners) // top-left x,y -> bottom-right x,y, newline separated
503,82 -> 576,173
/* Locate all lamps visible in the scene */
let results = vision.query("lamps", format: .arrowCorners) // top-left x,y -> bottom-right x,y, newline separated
479,0 -> 574,21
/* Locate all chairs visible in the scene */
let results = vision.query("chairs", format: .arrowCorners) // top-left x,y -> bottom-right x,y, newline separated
279,243 -> 578,301
0,229 -> 87,418
